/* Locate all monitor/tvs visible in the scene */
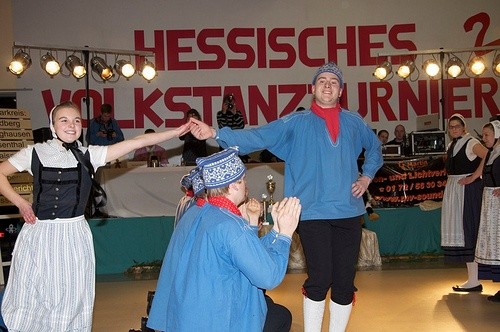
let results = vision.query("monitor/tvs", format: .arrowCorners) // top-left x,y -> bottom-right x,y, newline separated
412,131 -> 447,154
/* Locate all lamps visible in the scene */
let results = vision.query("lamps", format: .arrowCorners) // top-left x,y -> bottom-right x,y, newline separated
395,55 -> 420,82
492,50 -> 500,76
421,54 -> 442,81
372,56 -> 394,82
465,51 -> 488,77
60,51 -> 87,81
90,51 -> 115,84
113,55 -> 136,81
444,52 -> 465,78
137,57 -> 159,83
39,49 -> 61,79
6,47 -> 32,79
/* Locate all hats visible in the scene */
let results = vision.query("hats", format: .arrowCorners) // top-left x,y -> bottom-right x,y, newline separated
312,63 -> 343,86
179,145 -> 246,194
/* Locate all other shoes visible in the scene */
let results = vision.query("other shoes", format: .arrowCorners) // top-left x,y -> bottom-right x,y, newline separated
452,283 -> 482,292
368,213 -> 379,221
360,217 -> 365,225
488,291 -> 500,302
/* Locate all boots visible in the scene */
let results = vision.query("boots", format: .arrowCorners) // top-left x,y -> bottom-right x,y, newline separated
329,298 -> 352,332
303,294 -> 326,332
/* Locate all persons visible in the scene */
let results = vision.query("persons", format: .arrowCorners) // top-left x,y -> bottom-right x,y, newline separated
258,107 -> 306,162
134,128 -> 170,165
192,62 -> 383,332
179,109 -> 206,166
216,93 -> 248,164
86,104 -> 124,146
0,104 -> 179,330
472,120 -> 500,302
441,113 -> 489,292
175,167 -> 261,239
378,130 -> 388,143
382,125 -> 411,156
143,146 -> 301,331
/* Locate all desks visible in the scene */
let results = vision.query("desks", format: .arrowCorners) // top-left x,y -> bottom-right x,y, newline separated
363,207 -> 441,256
86,217 -> 175,275
96,162 -> 284,217
